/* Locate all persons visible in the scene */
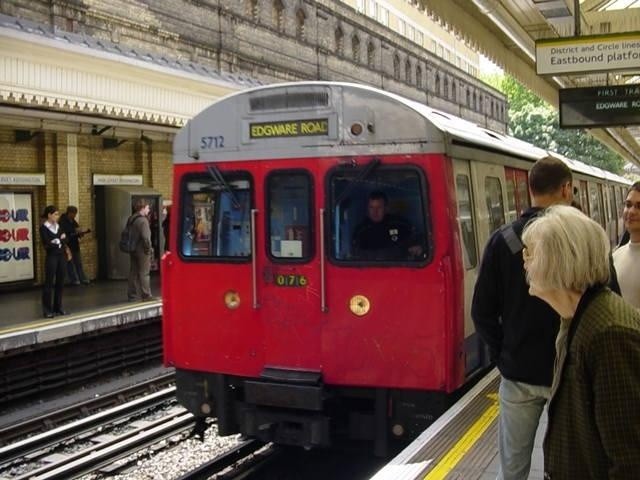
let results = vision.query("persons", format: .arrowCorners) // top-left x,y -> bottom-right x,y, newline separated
611,181 -> 639,310
57,206 -> 90,286
125,199 -> 159,302
38,205 -> 69,319
520,204 -> 639,479
470,157 -> 622,479
344,192 -> 427,263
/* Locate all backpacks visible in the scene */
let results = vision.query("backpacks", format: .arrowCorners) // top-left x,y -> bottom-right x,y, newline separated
119,215 -> 147,253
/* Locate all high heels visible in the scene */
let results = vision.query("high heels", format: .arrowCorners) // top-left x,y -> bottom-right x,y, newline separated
43,306 -> 67,318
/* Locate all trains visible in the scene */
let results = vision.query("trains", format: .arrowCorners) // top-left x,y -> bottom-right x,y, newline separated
159,81 -> 634,458
